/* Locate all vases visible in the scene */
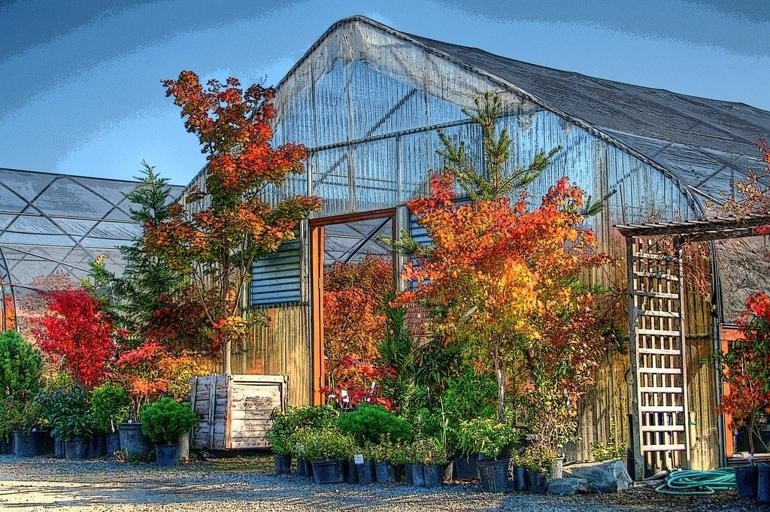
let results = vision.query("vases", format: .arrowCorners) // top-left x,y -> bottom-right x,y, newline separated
758,465 -> 770,504
735,465 -> 759,500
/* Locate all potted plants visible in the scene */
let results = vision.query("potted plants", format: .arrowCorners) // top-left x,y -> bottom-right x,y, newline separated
264,366 -> 583,495
0,328 -> 197,468
